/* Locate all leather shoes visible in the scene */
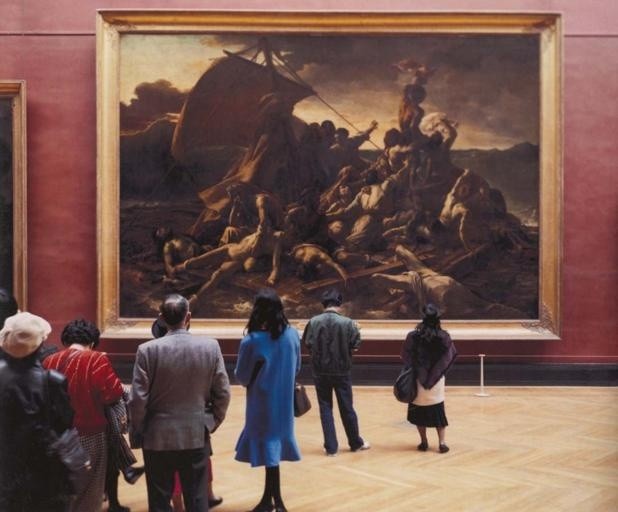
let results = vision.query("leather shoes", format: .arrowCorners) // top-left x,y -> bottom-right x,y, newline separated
439,444 -> 448,451
208,496 -> 222,507
418,443 -> 427,450
125,467 -> 143,484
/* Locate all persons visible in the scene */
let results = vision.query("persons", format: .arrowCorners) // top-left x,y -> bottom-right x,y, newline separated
301,285 -> 370,454
0,287 -> 232,512
399,301 -> 458,455
232,285 -> 304,512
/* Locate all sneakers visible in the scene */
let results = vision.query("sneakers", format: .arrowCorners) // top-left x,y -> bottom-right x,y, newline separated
355,443 -> 370,451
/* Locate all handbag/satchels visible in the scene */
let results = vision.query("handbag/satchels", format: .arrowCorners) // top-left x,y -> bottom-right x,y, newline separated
43,428 -> 92,493
394,368 -> 416,402
293,384 -> 311,417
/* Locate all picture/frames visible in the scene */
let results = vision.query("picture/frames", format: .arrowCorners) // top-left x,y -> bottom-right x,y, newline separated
1,78 -> 30,315
91,10 -> 565,344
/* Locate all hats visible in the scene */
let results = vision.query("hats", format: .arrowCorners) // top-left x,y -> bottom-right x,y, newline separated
0,312 -> 52,358
